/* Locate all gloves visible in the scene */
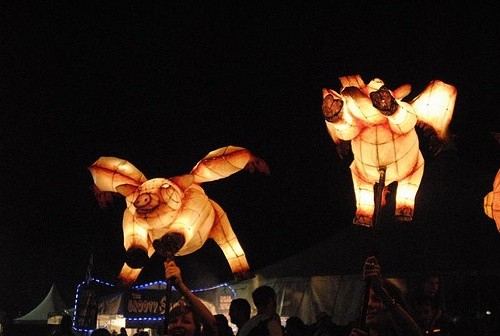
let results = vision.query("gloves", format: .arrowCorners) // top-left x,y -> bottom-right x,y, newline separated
363,262 -> 381,291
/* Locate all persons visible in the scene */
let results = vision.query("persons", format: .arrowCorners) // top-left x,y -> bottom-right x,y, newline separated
51,313 -> 150,336
202,259 -> 500,336
162,261 -> 220,336
